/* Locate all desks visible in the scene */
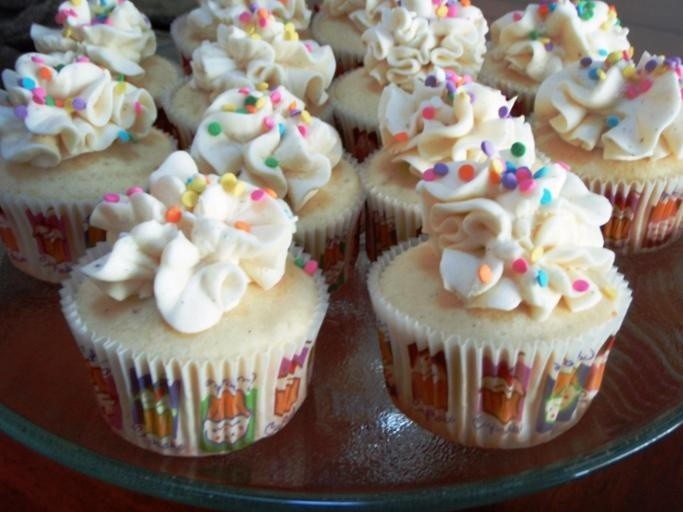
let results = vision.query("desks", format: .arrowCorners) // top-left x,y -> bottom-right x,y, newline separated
0,208 -> 681,511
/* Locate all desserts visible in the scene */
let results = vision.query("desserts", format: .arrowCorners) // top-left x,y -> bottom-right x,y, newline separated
1,0 -> 683,457
